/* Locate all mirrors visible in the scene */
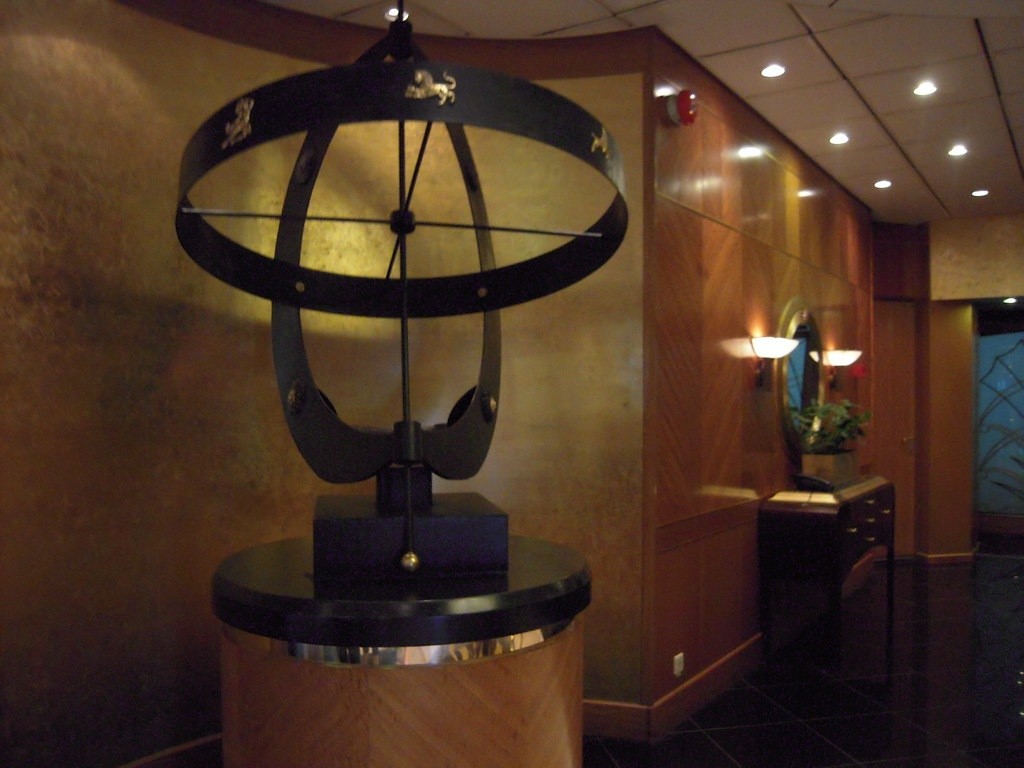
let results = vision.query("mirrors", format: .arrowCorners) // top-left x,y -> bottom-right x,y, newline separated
771,296 -> 824,467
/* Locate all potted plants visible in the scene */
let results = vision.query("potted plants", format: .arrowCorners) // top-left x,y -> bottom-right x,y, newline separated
790,398 -> 873,487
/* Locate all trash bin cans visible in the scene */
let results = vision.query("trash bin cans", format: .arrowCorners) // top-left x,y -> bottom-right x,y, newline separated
960,514 -> 1023,700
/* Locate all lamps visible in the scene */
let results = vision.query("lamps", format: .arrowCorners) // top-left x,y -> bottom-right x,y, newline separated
750,337 -> 800,392
827,349 -> 863,389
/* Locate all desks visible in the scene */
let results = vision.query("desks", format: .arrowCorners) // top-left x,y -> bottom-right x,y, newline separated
757,477 -> 895,671
